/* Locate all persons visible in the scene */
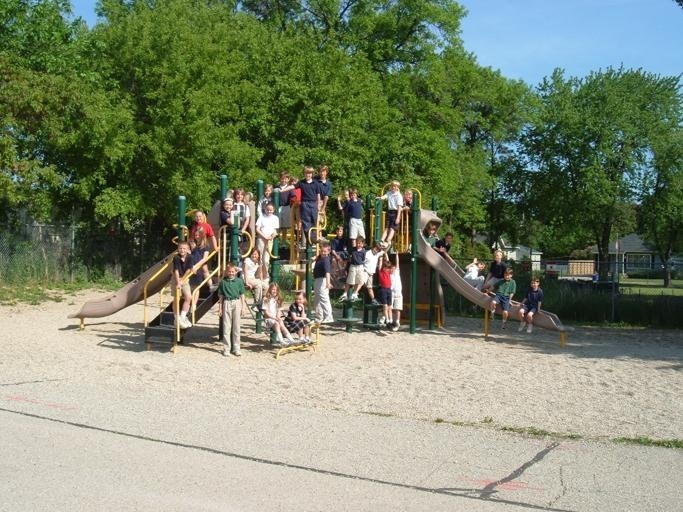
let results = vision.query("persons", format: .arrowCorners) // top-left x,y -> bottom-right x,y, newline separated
463,257 -> 487,292
489,268 -> 516,330
436,233 -> 453,258
517,278 -> 544,334
484,250 -> 508,290
174,165 -> 413,357
423,223 -> 446,253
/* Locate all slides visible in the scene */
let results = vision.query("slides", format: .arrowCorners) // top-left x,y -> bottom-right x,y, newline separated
417,211 -> 563,332
66,200 -> 223,318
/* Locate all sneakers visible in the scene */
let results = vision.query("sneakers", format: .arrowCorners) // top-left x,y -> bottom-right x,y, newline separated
251,304 -> 260,313
489,313 -> 494,321
501,320 -> 507,331
280,338 -> 314,348
339,291 -> 360,302
385,320 -> 402,332
173,318 -> 187,329
518,321 -> 527,332
378,239 -> 384,248
231,349 -> 241,356
370,298 -> 381,306
526,324 -> 533,333
383,242 -> 388,248
181,316 -> 192,327
188,285 -> 220,313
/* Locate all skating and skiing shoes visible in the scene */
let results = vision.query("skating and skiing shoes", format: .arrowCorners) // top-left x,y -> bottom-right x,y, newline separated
223,348 -> 230,356
